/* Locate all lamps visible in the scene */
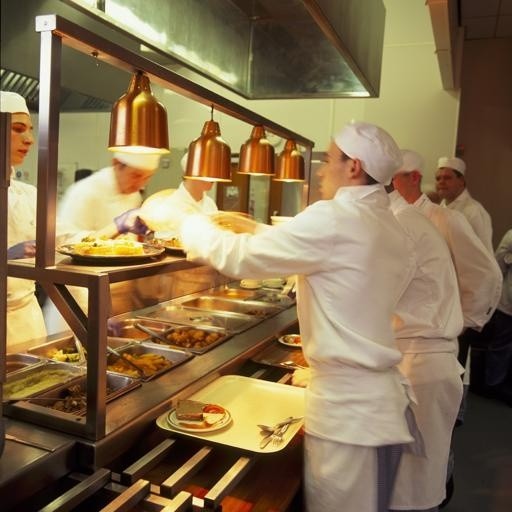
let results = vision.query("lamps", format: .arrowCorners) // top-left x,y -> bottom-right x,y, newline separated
177,101 -> 234,186
265,135 -> 306,185
235,123 -> 277,176
105,65 -> 172,157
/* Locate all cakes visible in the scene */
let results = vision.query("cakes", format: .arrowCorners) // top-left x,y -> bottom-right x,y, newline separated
74,236 -> 145,255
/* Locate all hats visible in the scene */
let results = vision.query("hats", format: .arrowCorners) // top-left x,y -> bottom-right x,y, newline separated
333,120 -> 405,186
438,156 -> 467,176
0,89 -> 31,116
112,146 -> 162,169
395,149 -> 426,176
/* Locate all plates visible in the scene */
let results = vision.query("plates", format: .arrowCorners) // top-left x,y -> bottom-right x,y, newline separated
157,242 -> 183,253
55,240 -> 166,264
278,333 -> 302,348
167,407 -> 233,433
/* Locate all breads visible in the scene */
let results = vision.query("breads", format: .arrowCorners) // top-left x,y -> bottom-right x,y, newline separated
176,399 -> 202,418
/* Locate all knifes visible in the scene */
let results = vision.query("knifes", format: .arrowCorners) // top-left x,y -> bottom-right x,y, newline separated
259,416 -> 294,449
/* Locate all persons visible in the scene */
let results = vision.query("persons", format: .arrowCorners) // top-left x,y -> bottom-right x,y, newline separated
140,121 -> 414,511
140,149 -> 213,222
433,158 -> 493,425
481,225 -> 510,401
43,146 -> 170,333
211,187 -> 463,510
0,90 -> 47,353
390,151 -> 503,331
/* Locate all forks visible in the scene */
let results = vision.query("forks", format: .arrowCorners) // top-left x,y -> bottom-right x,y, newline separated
272,421 -> 288,446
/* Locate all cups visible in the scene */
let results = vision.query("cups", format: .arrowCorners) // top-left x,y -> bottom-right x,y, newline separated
239,278 -> 284,290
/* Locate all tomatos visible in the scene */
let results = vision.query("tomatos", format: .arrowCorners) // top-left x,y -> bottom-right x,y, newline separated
203,405 -> 224,413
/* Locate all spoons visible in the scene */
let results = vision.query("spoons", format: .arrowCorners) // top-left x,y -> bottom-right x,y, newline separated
257,415 -> 303,432
280,359 -> 308,371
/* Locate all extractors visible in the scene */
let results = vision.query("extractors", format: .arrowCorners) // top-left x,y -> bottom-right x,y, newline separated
0,0 -> 389,118
